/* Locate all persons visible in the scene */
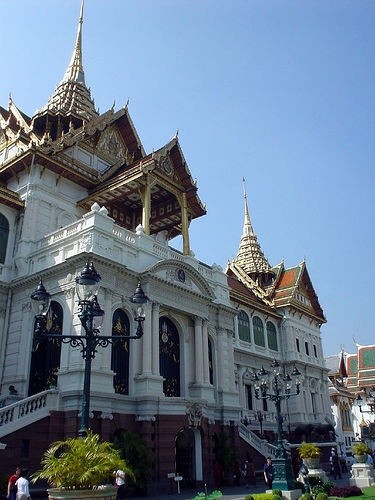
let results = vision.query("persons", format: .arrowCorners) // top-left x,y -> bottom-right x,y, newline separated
9,386 -> 17,395
15,469 -> 31,500
264,457 -> 274,489
332,450 -> 342,480
213,458 -> 260,489
297,458 -> 310,495
6,467 -> 21,500
366,449 -> 375,465
352,455 -> 357,464
341,451 -> 348,473
348,461 -> 352,472
113,469 -> 125,500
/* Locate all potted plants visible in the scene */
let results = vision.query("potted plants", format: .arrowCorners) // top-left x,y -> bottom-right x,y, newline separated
296,440 -> 322,468
28,424 -> 135,500
351,442 -> 369,463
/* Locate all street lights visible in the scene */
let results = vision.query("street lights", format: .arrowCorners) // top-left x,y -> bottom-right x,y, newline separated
29,258 -> 149,442
250,357 -> 304,500
